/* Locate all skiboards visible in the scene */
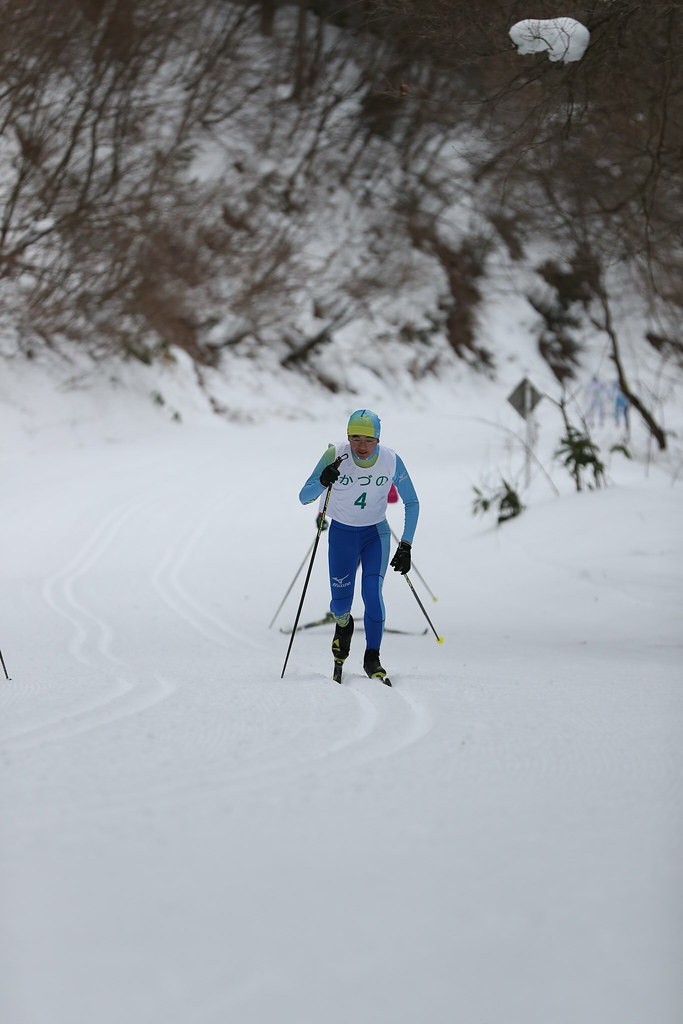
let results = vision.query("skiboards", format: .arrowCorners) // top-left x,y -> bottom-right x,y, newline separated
279,614 -> 430,638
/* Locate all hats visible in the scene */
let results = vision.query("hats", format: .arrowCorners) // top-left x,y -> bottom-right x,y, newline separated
347,409 -> 381,440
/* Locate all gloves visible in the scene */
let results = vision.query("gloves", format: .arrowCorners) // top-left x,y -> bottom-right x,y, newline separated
316,515 -> 329,530
320,457 -> 342,487
389,542 -> 411,575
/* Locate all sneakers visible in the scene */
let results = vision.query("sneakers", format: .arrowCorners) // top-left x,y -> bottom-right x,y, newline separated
332,614 -> 354,660
364,650 -> 386,679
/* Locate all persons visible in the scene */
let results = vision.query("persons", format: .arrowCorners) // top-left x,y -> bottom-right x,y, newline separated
299,409 -> 420,678
315,481 -> 398,615
